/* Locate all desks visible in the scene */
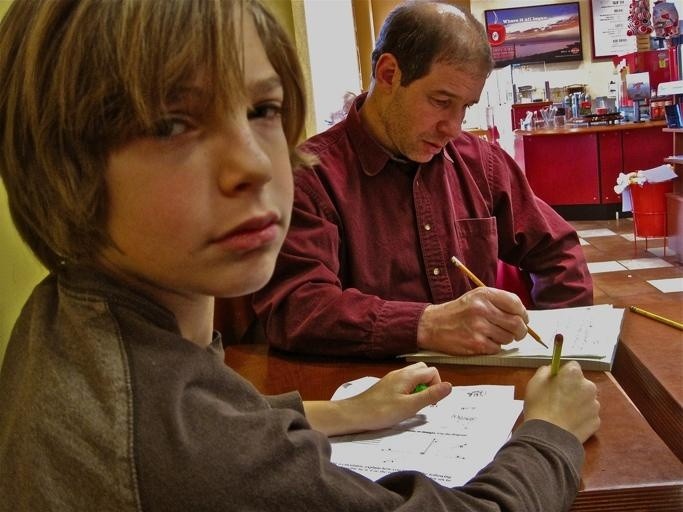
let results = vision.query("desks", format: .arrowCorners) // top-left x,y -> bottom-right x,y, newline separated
216,338 -> 682,512
614,314 -> 683,459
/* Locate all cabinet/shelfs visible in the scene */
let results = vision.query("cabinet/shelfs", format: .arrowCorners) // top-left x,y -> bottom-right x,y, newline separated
513,119 -> 683,205
662,126 -> 683,262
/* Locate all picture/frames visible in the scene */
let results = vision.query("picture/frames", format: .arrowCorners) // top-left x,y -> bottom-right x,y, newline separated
589,0 -> 652,61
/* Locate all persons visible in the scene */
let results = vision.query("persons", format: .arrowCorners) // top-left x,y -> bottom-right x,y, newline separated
253,1 -> 593,362
0,0 -> 601,512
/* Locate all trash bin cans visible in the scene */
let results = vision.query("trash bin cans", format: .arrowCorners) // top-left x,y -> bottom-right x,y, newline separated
629,180 -> 673,258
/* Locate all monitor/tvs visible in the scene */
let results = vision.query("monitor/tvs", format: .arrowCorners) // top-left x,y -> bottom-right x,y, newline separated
484,2 -> 583,68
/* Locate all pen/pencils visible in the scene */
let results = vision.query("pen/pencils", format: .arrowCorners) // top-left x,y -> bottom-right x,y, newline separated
550,334 -> 563,377
450,256 -> 548,349
628,304 -> 683,329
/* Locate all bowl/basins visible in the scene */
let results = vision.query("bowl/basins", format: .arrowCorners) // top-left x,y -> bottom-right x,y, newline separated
595,108 -> 608,116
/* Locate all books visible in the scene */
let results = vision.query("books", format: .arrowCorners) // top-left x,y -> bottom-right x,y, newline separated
397,306 -> 627,372
